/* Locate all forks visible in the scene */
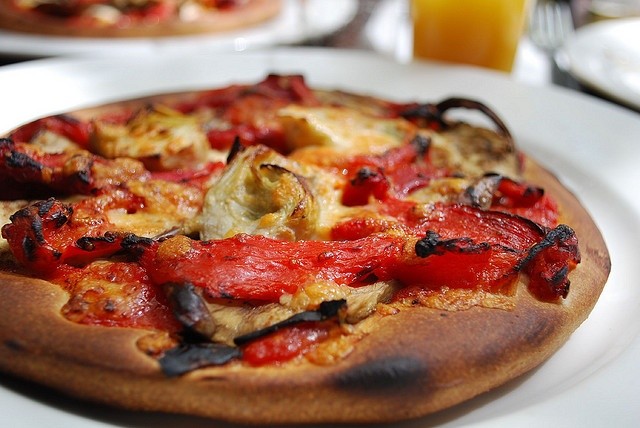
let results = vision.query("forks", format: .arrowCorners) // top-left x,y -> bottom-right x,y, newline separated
529,2 -> 637,105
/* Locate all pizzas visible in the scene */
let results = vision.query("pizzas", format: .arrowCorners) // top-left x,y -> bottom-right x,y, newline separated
1,0 -> 281,36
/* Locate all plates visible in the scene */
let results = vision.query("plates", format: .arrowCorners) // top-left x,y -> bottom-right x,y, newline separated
1,0 -> 360,58
